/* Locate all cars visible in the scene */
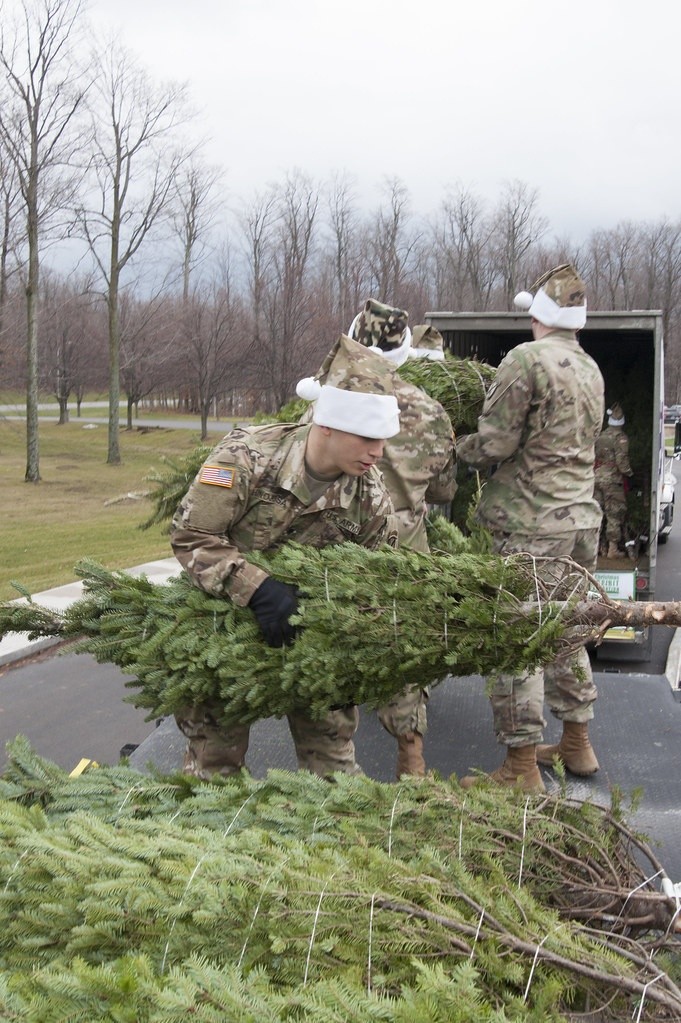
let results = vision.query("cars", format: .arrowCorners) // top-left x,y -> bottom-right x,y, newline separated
664,405 -> 681,423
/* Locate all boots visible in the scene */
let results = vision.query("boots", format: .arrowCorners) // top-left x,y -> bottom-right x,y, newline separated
535,717 -> 601,775
606,542 -> 626,558
599,537 -> 608,558
459,743 -> 547,795
392,733 -> 425,781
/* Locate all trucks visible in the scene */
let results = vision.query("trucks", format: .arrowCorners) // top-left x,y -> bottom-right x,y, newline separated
415,307 -> 678,661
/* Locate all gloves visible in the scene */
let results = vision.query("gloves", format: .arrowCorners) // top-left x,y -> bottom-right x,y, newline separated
248,578 -> 305,648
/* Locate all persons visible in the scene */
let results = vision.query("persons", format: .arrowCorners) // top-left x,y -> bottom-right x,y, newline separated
347,298 -> 444,778
592,403 -> 633,559
451,265 -> 606,796
169,333 -> 399,780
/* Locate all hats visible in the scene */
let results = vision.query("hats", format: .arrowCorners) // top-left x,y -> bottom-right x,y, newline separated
410,323 -> 446,360
606,402 -> 625,426
296,334 -> 401,439
346,299 -> 412,372
514,264 -> 588,330
